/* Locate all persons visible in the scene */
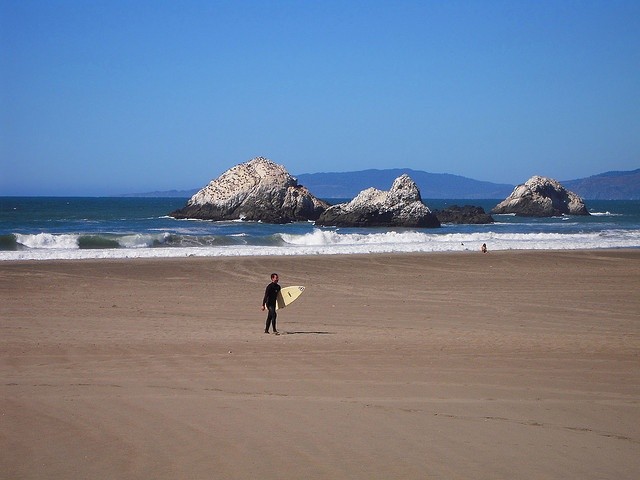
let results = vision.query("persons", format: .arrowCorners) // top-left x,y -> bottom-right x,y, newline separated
262,274 -> 284,335
482,243 -> 488,253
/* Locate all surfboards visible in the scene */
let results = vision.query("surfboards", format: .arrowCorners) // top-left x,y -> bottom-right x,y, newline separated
265,286 -> 305,310
